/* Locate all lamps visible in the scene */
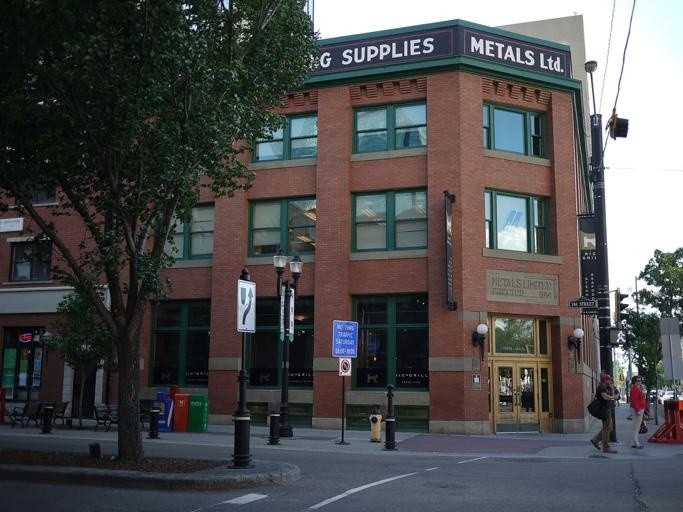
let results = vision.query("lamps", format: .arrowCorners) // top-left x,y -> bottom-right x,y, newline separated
473,323 -> 488,361
568,327 -> 585,365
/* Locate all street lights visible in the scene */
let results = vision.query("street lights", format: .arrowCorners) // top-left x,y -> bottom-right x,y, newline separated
271,248 -> 305,438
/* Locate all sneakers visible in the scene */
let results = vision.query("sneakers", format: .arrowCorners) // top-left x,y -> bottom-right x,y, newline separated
591,439 -> 617,453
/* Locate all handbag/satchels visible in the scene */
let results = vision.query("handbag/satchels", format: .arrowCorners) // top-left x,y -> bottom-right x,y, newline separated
587,398 -> 608,420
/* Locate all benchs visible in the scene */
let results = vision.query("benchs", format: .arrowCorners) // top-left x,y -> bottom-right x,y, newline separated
93,402 -> 145,431
9,401 -> 69,426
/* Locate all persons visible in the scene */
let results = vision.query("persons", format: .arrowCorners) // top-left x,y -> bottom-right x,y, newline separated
630,376 -> 646,449
613,386 -> 620,406
590,372 -> 617,453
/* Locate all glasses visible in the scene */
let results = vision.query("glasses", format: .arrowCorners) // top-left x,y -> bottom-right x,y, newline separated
636,380 -> 641,382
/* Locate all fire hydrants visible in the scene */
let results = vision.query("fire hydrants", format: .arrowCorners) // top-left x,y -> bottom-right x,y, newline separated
368,407 -> 382,443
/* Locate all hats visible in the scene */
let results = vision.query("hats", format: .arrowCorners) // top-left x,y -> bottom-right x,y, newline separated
601,374 -> 613,379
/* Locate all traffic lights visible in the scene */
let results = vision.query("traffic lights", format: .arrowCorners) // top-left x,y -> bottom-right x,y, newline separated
614,288 -> 629,324
